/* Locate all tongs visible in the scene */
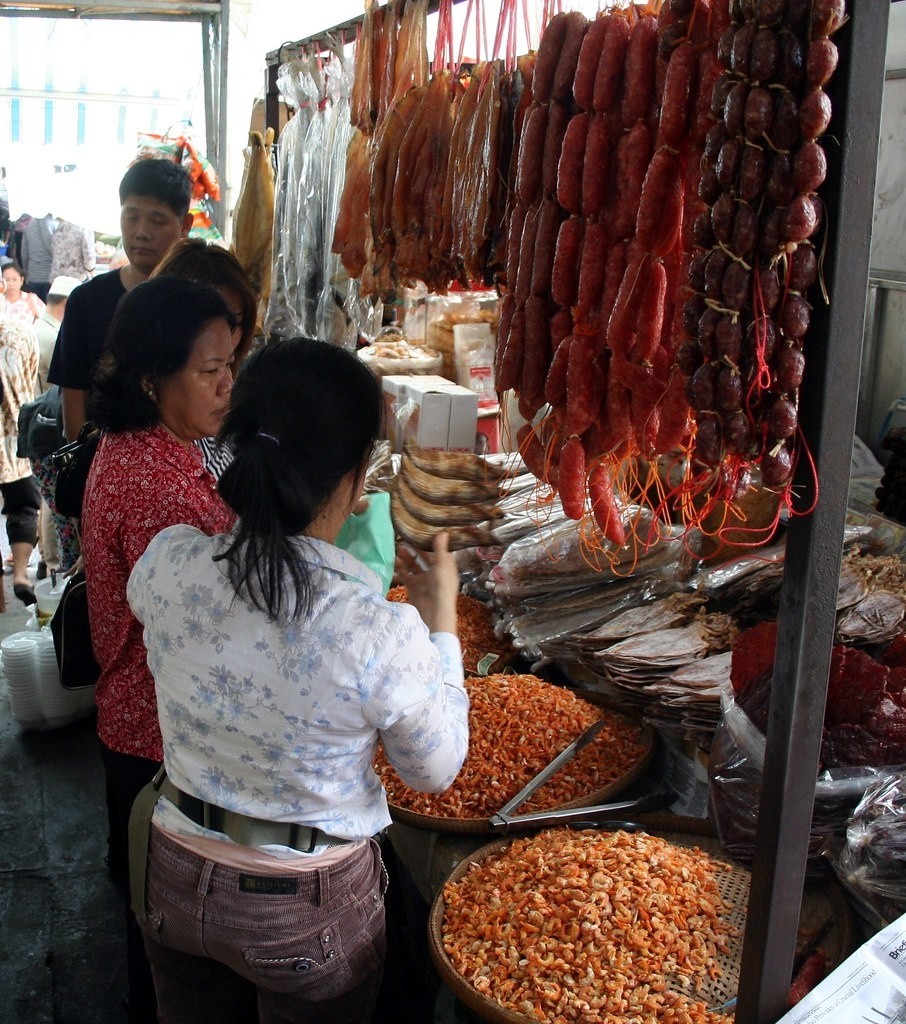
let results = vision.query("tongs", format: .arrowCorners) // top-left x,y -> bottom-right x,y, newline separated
489,715 -> 680,831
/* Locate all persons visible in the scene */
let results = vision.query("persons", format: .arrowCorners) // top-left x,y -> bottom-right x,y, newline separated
122,338 -> 471,1023
1,254 -> 83,609
41,157 -> 197,447
145,238 -> 258,484
77,273 -> 251,981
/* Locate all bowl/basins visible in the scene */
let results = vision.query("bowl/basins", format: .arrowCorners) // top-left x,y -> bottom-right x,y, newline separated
2,620 -> 81,727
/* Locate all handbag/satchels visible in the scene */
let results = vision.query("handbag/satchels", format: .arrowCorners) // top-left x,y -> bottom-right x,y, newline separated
17,385 -> 60,459
50,572 -> 101,690
55,422 -> 106,518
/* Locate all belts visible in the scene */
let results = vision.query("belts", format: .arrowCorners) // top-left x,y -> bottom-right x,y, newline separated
128,763 -> 342,922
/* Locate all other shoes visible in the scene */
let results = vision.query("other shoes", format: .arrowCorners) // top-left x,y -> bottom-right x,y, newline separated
0,554 -> 46,605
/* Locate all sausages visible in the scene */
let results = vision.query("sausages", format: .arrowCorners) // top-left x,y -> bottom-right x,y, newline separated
508,3 -> 843,562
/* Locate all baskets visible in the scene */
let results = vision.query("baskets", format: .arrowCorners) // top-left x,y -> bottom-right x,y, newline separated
388,689 -> 658,834
427,812 -> 750,1024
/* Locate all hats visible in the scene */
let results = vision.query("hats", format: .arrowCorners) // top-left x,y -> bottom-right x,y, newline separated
48,276 -> 82,296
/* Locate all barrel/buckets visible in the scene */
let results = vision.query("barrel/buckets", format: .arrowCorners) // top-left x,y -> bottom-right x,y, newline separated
35,575 -> 72,622
